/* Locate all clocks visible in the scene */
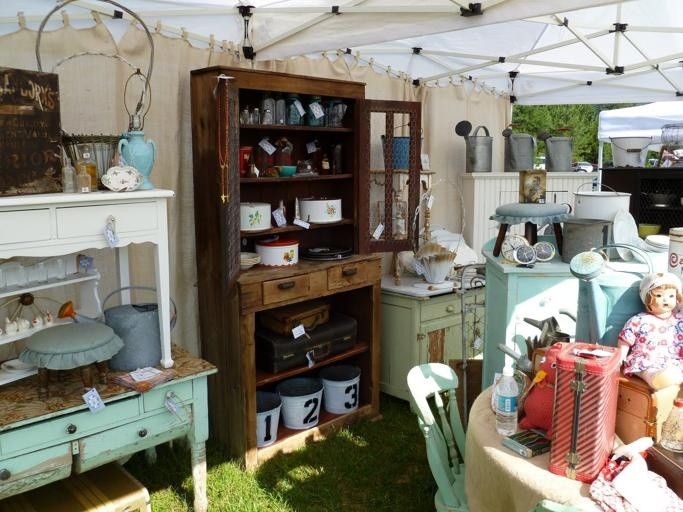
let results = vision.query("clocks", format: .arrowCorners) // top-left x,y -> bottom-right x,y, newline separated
512,242 -> 537,269
530,240 -> 557,263
497,231 -> 531,266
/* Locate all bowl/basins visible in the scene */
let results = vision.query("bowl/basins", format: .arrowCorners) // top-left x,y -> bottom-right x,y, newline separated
240,251 -> 262,272
276,165 -> 297,177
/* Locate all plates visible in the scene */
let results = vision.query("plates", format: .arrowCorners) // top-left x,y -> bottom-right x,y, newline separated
644,233 -> 669,253
101,165 -> 144,191
300,245 -> 353,262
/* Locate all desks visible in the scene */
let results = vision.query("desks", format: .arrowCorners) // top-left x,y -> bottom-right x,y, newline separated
0,184 -> 181,373
0,337 -> 223,512
460,379 -> 603,512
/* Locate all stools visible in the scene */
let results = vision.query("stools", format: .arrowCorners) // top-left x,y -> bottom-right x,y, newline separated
16,318 -> 128,404
486,202 -> 570,260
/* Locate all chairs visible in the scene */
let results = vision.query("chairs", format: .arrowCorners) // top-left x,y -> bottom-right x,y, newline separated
400,358 -> 471,512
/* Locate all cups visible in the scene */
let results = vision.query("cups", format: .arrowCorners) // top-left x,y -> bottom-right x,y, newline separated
667,227 -> 682,279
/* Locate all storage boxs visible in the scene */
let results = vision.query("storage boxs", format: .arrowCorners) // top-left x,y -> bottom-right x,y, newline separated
542,339 -> 623,485
251,311 -> 360,375
260,299 -> 333,338
0,459 -> 154,512
613,373 -> 659,446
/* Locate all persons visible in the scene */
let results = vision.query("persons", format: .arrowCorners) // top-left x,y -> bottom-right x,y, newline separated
618,271 -> 683,441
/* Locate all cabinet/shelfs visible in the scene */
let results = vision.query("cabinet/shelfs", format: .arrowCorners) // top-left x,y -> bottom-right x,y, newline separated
476,247 -> 649,393
597,165 -> 682,242
184,62 -> 427,474
376,266 -> 486,417
0,249 -> 113,400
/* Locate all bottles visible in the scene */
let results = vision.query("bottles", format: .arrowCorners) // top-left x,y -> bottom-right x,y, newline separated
494,366 -> 518,435
62,144 -> 96,194
238,94 -> 344,128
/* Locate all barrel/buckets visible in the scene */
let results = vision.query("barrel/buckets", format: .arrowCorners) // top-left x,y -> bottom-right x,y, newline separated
573,181 -> 633,259
560,218 -> 614,269
382,122 -> 425,171
609,134 -> 653,167
255,391 -> 285,447
277,377 -> 325,431
322,364 -> 362,416
